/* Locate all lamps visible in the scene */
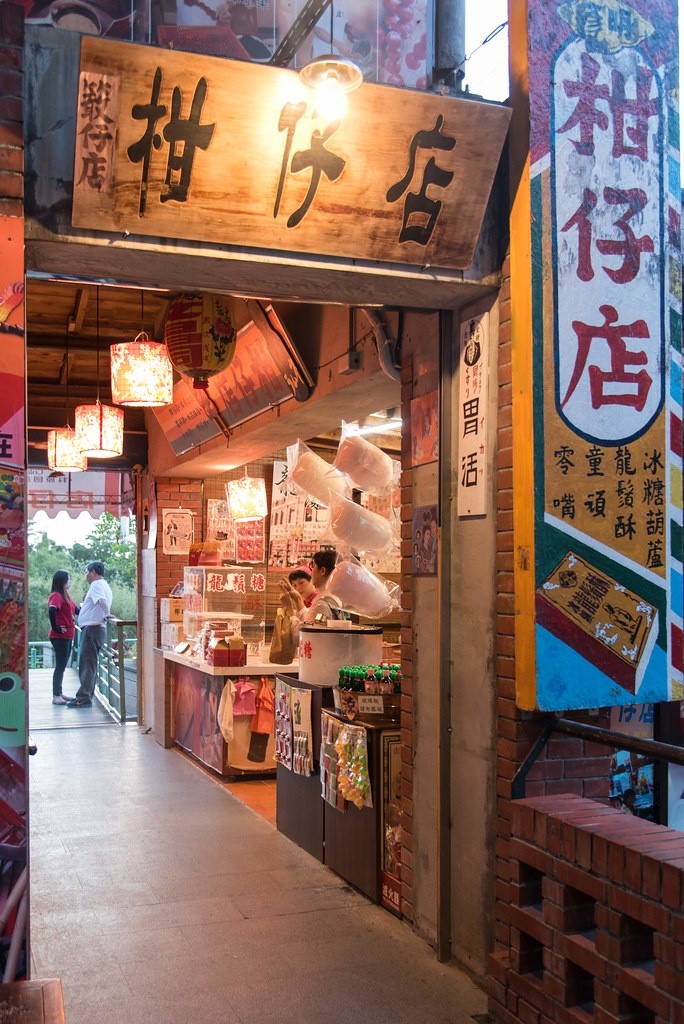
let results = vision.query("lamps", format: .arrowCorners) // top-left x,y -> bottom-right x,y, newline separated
47,315 -> 89,473
225,465 -> 268,522
75,285 -> 125,458
299,0 -> 362,97
108,288 -> 173,409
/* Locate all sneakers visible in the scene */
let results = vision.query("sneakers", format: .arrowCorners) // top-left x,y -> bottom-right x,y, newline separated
67,698 -> 92,708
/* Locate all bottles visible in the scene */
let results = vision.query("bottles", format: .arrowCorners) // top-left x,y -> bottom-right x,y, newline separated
338,661 -> 403,695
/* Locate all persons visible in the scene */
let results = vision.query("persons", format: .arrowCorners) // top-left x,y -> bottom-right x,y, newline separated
276,551 -> 345,627
112,632 -> 129,659
48,562 -> 115,709
611,789 -> 636,815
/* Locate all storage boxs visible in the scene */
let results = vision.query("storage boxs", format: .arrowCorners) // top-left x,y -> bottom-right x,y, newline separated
203,622 -> 247,668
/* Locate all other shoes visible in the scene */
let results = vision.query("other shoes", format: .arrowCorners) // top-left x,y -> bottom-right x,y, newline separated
52,694 -> 74,705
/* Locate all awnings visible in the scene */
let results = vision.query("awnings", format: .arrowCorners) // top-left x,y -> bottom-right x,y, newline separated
27,469 -> 136,519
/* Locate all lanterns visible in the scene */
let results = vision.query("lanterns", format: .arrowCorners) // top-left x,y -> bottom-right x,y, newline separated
165,291 -> 237,389
